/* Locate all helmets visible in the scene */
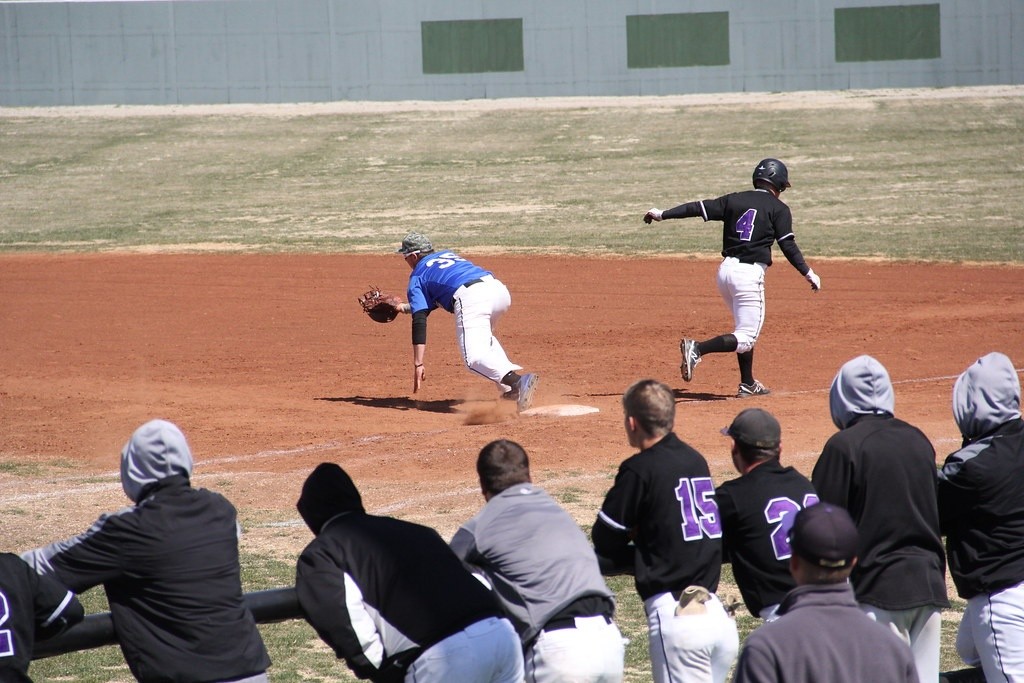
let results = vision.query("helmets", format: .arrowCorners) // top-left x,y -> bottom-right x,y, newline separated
752,158 -> 791,193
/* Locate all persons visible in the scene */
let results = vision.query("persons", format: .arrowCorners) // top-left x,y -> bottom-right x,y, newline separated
295,438 -> 625,682
590,351 -> 1024,683
0,415 -> 277,683
642,157 -> 821,398
357,235 -> 541,416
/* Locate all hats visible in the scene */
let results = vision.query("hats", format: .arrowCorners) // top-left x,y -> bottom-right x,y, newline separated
398,232 -> 435,254
788,501 -> 861,570
721,408 -> 782,449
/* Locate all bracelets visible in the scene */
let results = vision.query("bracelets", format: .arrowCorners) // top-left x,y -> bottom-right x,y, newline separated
415,363 -> 423,368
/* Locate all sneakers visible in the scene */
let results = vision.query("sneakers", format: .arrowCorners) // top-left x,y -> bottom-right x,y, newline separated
680,338 -> 701,383
500,372 -> 539,413
737,379 -> 771,398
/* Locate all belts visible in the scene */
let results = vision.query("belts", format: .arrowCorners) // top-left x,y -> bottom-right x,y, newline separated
544,614 -> 612,632
451,279 -> 484,308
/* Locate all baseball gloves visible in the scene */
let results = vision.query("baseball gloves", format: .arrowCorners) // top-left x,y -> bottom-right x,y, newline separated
357,290 -> 403,323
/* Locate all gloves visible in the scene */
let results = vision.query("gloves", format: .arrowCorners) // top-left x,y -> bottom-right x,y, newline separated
804,268 -> 821,292
643,208 -> 667,224
724,594 -> 745,617
675,584 -> 712,617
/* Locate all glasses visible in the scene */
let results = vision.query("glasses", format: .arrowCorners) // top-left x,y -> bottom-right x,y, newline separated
402,249 -> 421,259
